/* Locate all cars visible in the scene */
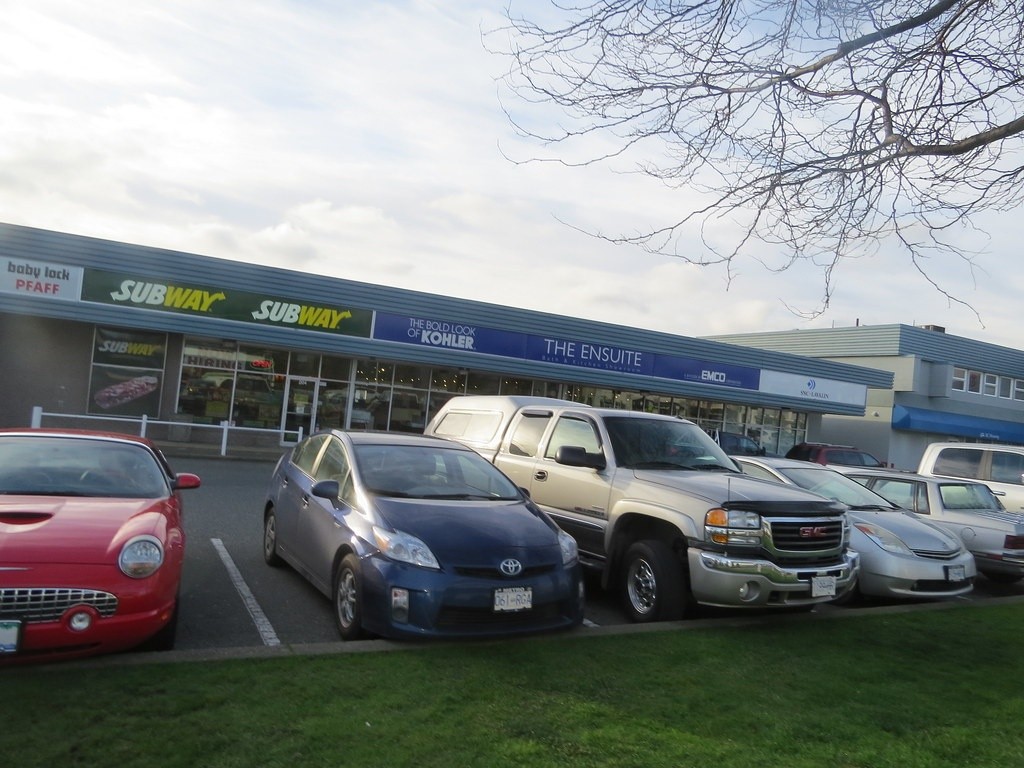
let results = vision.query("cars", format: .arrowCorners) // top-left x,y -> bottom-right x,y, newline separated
180,372 -> 435,436
263,430 -> 588,639
806,463 -> 1024,584
0,428 -> 201,657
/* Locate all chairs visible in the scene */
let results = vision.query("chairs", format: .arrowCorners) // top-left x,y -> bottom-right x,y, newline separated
409,457 -> 437,485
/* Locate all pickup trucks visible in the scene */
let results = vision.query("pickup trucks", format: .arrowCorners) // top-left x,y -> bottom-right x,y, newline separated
432,395 -> 861,627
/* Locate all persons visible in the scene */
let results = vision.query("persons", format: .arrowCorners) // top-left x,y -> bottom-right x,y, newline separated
661,430 -> 696,458
97,447 -> 137,489
358,394 -> 366,404
287,386 -> 295,413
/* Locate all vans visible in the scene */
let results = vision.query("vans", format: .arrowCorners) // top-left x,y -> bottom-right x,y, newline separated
915,443 -> 1024,513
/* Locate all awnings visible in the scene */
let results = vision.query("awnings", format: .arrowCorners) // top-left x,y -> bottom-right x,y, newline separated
892,405 -> 1024,444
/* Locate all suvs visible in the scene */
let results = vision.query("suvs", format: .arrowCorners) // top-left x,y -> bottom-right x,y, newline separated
784,443 -> 895,469
667,428 -> 786,464
691,450 -> 976,606
